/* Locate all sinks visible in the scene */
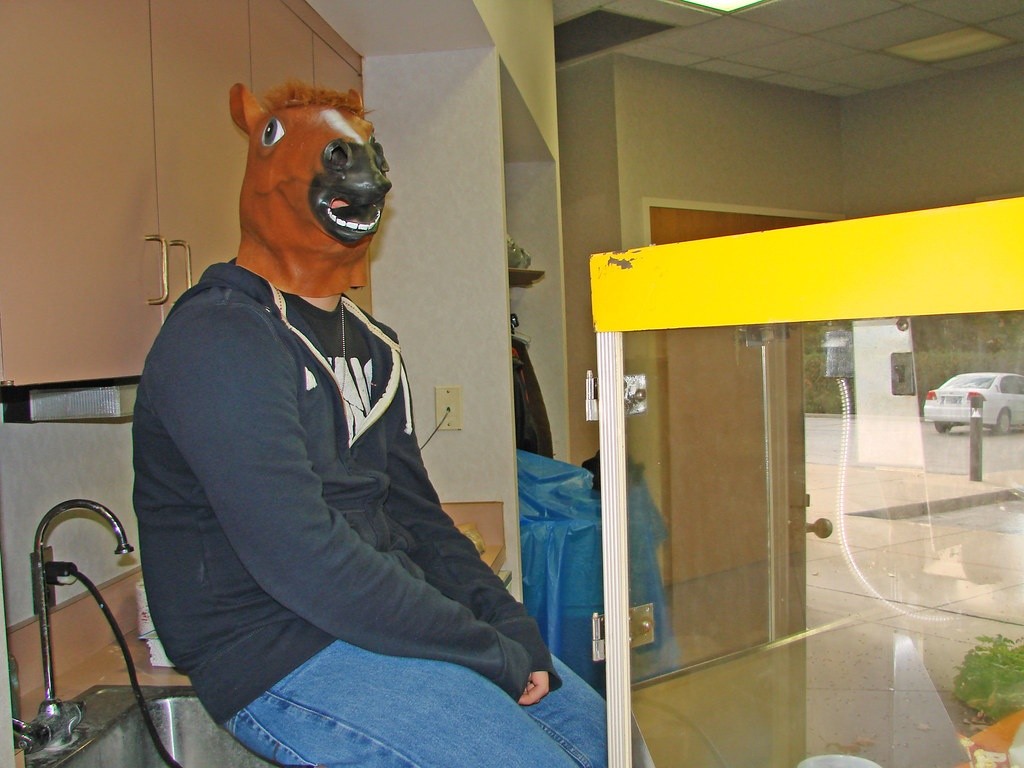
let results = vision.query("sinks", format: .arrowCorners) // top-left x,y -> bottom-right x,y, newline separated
13,685 -> 336,768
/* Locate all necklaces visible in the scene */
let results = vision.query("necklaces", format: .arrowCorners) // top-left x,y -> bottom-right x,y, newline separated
275,287 -> 346,399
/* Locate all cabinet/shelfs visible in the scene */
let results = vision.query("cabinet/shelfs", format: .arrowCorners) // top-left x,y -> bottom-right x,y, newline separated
1,0 -> 373,389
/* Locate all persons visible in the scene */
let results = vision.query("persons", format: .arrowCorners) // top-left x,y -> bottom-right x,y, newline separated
131,82 -> 608,768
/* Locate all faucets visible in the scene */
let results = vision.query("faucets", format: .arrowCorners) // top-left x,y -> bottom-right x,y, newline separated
34,499 -> 134,749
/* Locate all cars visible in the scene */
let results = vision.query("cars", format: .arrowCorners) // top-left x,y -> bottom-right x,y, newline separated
923,371 -> 1024,434
